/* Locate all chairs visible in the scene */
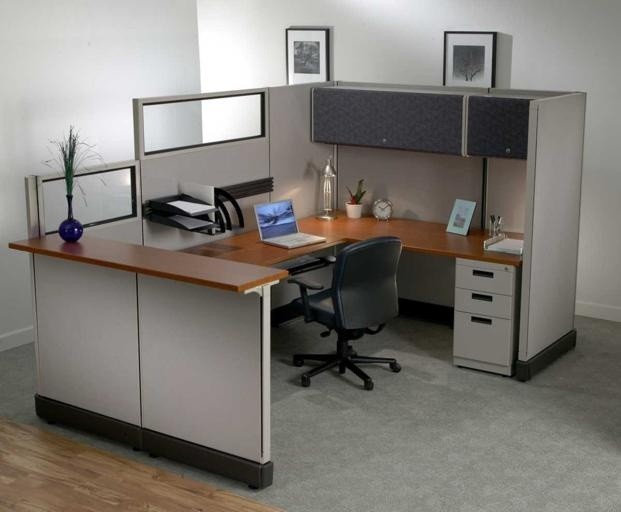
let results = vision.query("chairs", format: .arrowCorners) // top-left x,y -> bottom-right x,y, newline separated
288,238 -> 404,392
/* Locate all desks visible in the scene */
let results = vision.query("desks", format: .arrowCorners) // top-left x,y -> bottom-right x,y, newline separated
8,78 -> 586,491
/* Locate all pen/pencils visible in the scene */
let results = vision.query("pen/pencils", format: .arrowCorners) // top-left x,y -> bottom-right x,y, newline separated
491,214 -> 501,235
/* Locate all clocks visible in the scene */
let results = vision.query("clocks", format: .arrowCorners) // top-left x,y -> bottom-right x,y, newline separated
371,198 -> 392,224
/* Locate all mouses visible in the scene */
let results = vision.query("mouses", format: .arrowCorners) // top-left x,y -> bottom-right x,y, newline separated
325,255 -> 336,262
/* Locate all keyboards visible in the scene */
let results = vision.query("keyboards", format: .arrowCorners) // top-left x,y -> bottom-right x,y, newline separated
271,254 -> 320,270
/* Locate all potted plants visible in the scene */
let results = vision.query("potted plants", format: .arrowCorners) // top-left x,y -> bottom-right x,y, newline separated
50,125 -> 92,244
342,179 -> 368,220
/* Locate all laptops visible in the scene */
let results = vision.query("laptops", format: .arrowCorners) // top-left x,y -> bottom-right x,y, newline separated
254,199 -> 326,248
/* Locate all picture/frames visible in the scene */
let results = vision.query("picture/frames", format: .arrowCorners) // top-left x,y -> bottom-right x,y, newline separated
283,26 -> 330,87
440,30 -> 498,89
445,198 -> 477,237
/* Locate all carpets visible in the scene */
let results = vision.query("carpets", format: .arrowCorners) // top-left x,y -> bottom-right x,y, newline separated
0,314 -> 621,510
